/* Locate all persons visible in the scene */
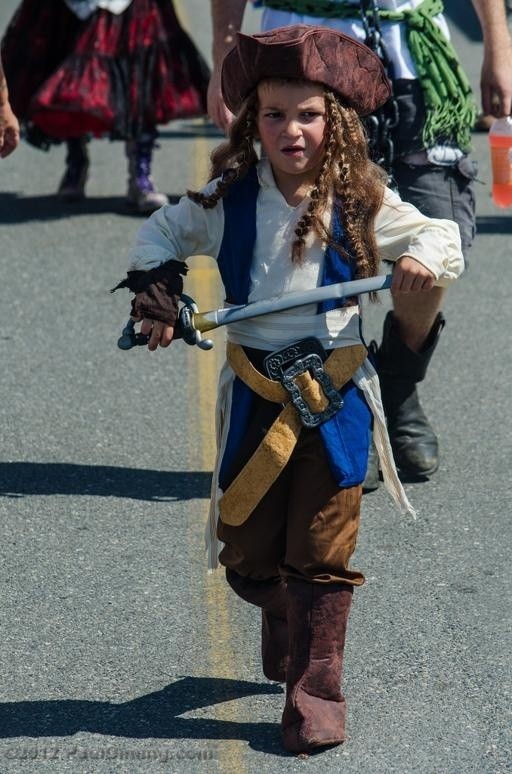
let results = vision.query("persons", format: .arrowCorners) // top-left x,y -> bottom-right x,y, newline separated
0,0 -> 211,213
203,1 -> 512,481
109,28 -> 466,754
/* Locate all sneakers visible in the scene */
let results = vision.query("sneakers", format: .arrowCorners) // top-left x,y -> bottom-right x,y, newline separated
56,160 -> 90,207
126,177 -> 172,210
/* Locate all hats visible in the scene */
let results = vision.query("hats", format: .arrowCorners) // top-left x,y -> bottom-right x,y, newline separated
220,24 -> 393,119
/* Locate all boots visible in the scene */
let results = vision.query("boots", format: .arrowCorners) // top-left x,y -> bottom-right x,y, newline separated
380,308 -> 446,478
281,579 -> 355,753
225,566 -> 290,683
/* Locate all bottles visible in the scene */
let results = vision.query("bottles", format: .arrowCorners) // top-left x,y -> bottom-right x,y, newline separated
488,114 -> 512,210
